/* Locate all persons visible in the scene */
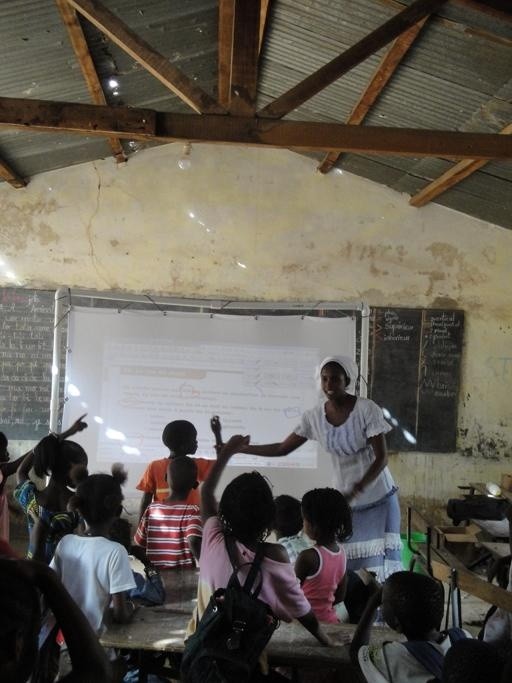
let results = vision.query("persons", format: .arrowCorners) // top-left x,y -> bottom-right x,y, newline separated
1,412 -> 146,683
214,356 -> 402,627
136,415 -> 475,683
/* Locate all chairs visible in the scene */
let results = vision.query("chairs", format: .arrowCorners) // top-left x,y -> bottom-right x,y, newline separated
431,560 -> 512,635
404,502 -> 475,588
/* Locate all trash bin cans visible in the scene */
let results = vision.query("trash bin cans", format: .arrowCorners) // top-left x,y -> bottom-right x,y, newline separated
399,531 -> 429,575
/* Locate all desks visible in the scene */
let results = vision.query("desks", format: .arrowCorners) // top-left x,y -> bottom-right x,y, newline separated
155,567 -> 201,612
103,609 -> 408,678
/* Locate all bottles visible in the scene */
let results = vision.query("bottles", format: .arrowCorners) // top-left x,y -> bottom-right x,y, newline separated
485,481 -> 501,495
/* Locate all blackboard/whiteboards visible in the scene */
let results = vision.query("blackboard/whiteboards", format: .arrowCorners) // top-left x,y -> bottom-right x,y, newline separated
0,286 -> 464,454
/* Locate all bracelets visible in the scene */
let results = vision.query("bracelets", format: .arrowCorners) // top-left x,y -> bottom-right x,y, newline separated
351,482 -> 364,499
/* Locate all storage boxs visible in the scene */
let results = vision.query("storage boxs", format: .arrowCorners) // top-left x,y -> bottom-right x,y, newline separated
436,521 -> 481,567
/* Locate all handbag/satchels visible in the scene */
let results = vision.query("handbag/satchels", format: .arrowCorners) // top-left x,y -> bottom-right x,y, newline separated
130,555 -> 165,607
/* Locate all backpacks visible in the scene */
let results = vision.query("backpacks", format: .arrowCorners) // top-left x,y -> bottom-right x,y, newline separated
401,627 -> 511,682
179,533 -> 280,683
